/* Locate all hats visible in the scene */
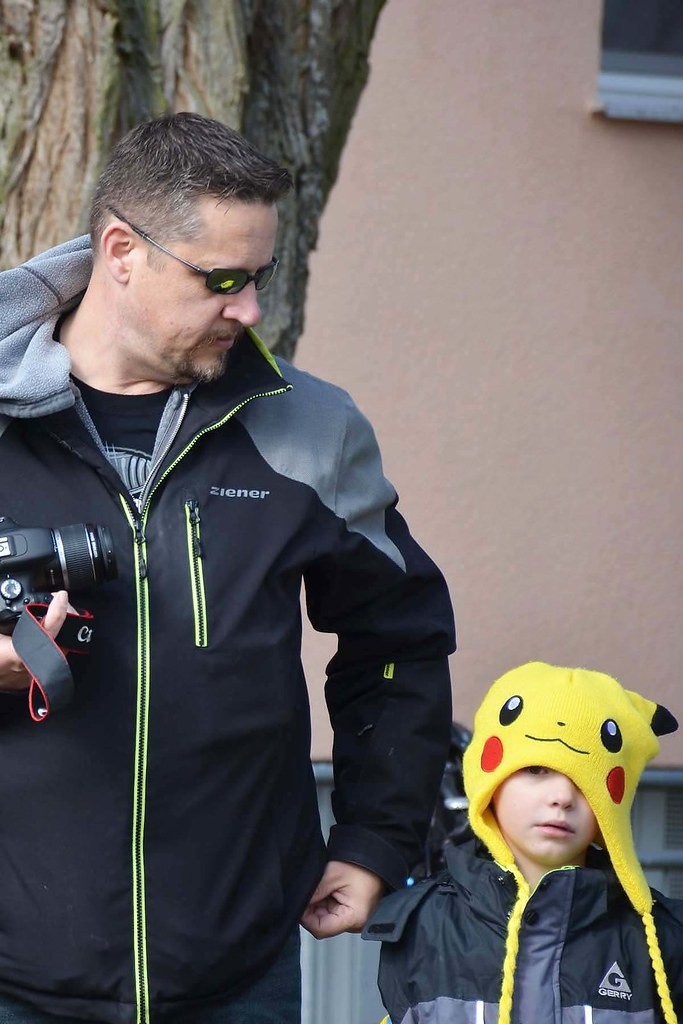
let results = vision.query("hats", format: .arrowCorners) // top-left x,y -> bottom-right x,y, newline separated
463,661 -> 678,1024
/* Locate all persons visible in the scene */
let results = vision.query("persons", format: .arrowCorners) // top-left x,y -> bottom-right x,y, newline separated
358,663 -> 683,1024
0,110 -> 460,1024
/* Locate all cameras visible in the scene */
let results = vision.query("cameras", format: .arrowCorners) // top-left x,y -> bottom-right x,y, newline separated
0,517 -> 118,636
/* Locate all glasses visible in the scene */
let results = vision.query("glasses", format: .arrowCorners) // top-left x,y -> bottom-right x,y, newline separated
107,204 -> 279,295
